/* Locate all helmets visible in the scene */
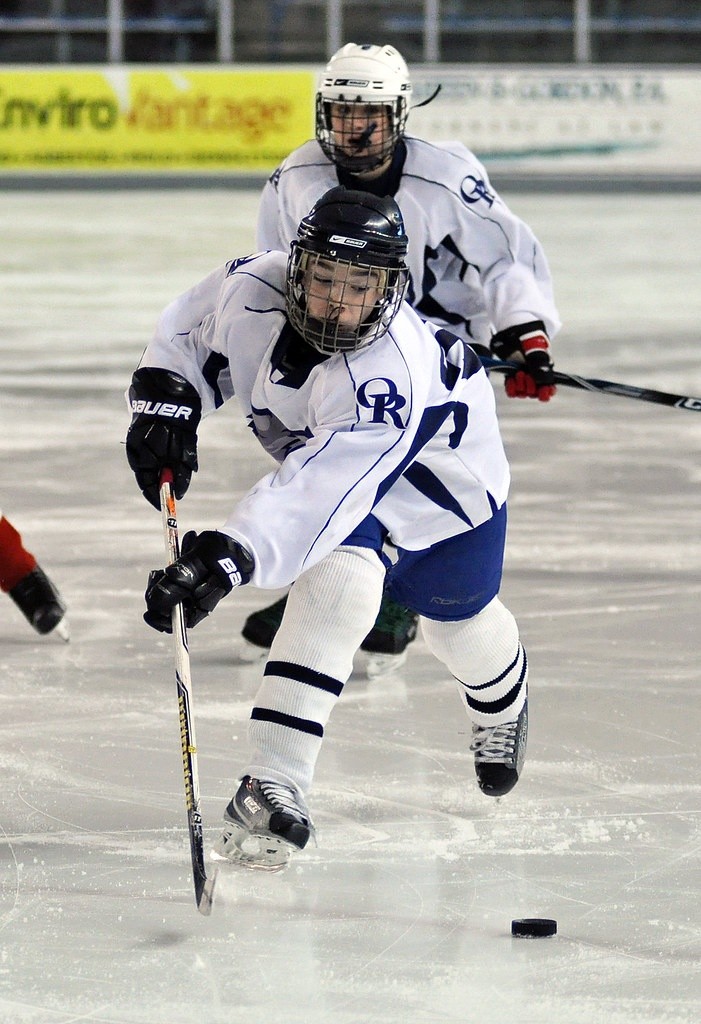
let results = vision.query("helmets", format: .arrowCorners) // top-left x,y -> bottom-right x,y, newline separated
313,42 -> 411,175
284,186 -> 411,355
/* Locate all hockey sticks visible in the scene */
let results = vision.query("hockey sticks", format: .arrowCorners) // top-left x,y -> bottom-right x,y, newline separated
157,467 -> 219,917
478,353 -> 701,413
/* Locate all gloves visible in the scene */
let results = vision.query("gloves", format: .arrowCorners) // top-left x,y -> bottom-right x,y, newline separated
144,531 -> 255,634
491,320 -> 557,402
125,368 -> 201,512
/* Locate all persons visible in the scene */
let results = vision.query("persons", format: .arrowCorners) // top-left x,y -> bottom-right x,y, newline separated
237,41 -> 563,679
1,514 -> 74,643
126,184 -> 531,874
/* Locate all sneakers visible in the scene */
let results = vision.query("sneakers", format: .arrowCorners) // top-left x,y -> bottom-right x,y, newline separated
236,591 -> 290,663
468,684 -> 531,806
210,779 -> 320,875
359,602 -> 421,681
7,566 -> 70,642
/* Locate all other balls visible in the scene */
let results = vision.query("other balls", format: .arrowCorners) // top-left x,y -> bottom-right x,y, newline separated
510,918 -> 558,938
